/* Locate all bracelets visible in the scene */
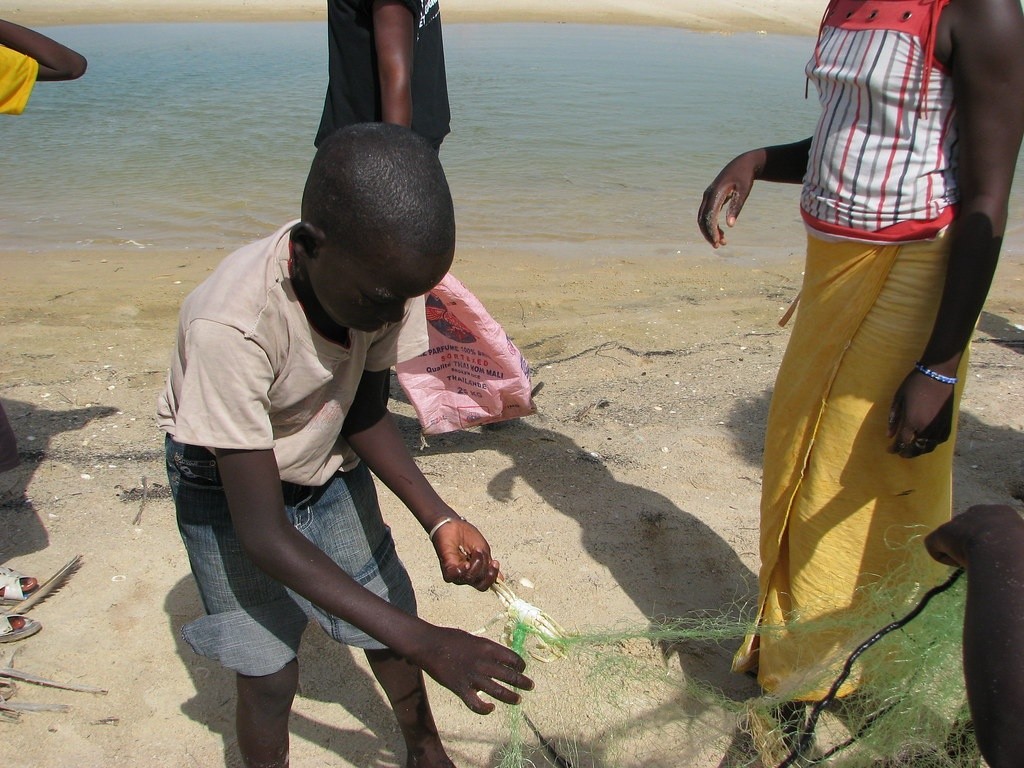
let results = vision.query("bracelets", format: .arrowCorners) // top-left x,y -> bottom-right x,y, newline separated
429,517 -> 467,540
915,362 -> 957,384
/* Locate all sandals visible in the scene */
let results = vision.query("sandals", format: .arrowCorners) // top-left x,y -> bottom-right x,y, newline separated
0,612 -> 42,643
0,565 -> 40,607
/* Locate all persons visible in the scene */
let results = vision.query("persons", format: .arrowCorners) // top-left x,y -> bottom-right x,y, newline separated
156,123 -> 534,767
699,1 -> 1024,750
0,21 -> 88,642
313,0 -> 451,154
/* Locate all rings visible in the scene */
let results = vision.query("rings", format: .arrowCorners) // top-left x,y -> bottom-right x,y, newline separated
915,438 -> 928,449
